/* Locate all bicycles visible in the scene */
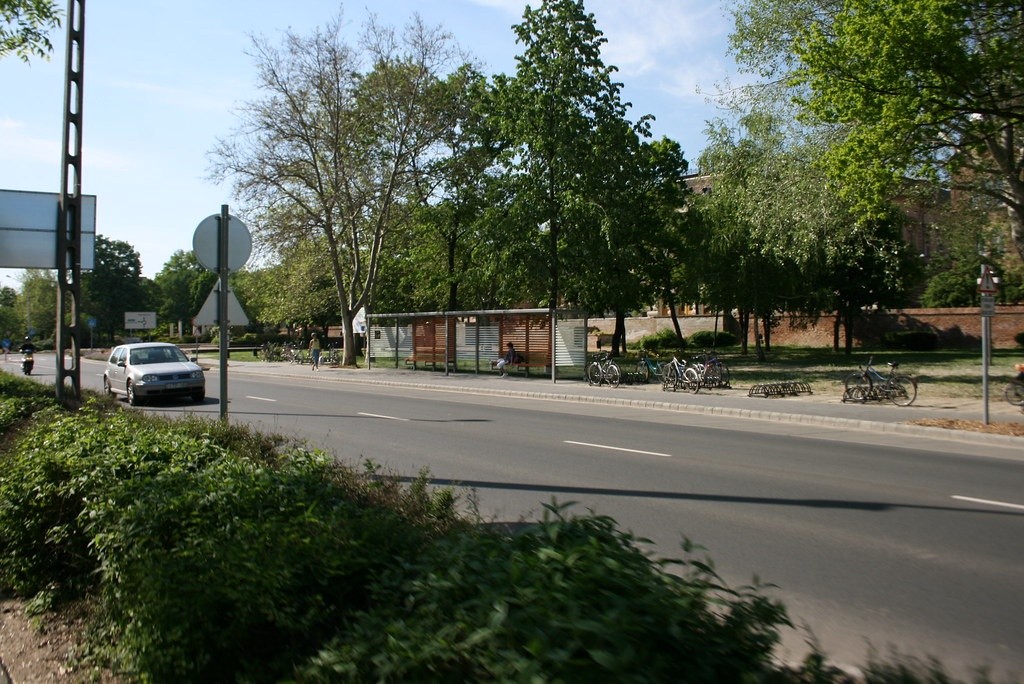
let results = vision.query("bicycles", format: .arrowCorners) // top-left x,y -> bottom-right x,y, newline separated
260,341 -> 339,365
583,350 -> 730,394
844,353 -> 918,407
1001,377 -> 1024,406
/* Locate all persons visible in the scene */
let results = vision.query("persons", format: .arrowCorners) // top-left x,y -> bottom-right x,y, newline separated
308,333 -> 320,371
1,334 -> 12,361
497,343 -> 515,378
20,336 -> 36,369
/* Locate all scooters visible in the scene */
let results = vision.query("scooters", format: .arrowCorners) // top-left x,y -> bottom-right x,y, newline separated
20,349 -> 36,375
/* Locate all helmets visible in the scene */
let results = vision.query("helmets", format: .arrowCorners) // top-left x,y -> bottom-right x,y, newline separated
25,336 -> 31,341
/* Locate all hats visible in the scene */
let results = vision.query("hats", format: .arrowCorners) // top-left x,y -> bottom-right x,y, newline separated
507,342 -> 513,348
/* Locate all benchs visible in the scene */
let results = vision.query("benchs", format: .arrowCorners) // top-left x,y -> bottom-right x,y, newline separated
489,355 -> 551,374
405,356 -> 456,372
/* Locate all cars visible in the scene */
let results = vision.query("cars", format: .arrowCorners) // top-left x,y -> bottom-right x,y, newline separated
104,342 -> 206,406
106,338 -> 142,348
